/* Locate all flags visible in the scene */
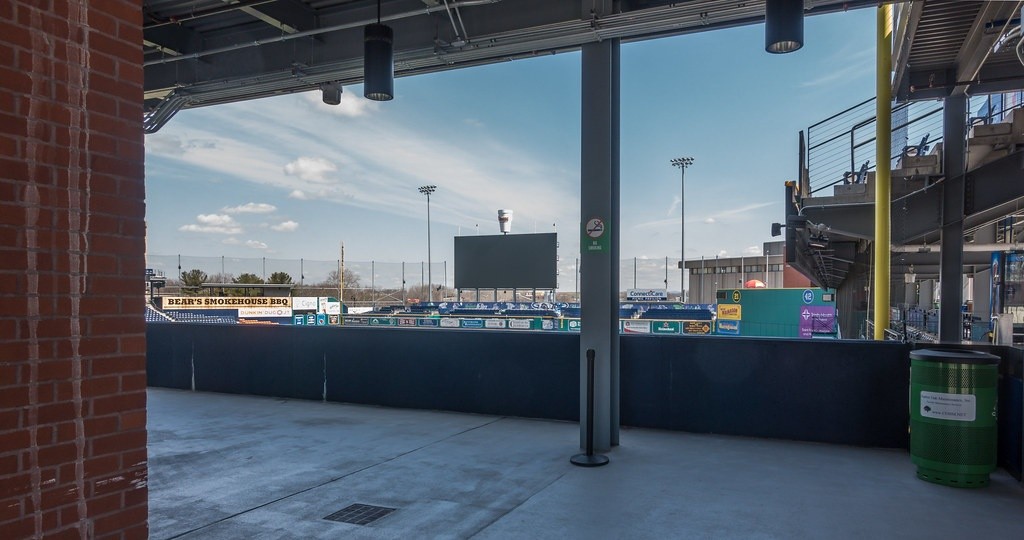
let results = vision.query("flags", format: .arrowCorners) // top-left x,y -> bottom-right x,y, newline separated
436,285 -> 441,291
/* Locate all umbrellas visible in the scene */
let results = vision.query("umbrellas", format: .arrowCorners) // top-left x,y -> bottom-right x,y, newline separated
744,279 -> 767,287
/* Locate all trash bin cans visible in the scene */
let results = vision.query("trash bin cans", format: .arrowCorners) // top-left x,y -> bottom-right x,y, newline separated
908,347 -> 1002,489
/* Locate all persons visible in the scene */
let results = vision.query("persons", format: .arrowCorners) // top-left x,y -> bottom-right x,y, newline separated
350,296 -> 356,307
248,290 -> 251,296
219,288 -> 229,297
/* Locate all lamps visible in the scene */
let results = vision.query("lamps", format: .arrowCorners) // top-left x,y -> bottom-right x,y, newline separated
766,0 -> 804,54
364,0 -> 394,101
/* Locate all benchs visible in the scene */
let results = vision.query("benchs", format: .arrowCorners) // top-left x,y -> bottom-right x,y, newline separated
843,104 -> 996,185
